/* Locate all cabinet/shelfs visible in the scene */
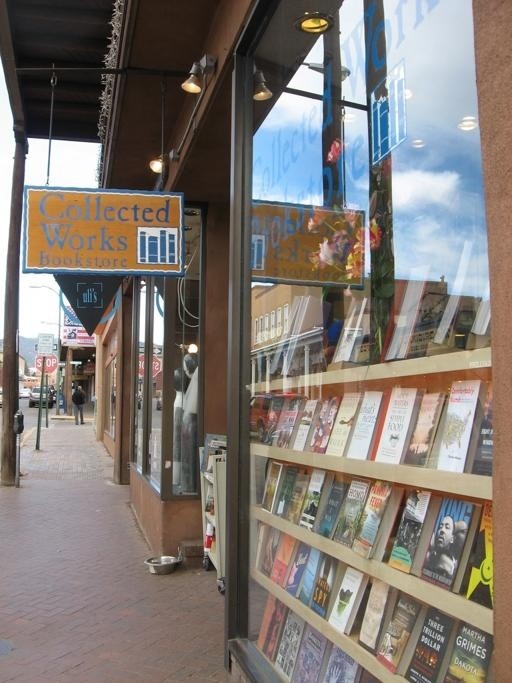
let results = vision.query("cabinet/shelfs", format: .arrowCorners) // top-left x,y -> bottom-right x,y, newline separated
244,347 -> 496,682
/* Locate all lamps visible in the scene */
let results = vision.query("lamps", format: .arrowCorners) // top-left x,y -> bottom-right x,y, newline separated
253,63 -> 274,102
148,148 -> 181,174
179,343 -> 199,354
177,51 -> 217,95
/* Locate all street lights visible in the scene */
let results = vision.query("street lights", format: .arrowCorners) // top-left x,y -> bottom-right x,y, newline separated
31,283 -> 67,306
40,320 -> 63,329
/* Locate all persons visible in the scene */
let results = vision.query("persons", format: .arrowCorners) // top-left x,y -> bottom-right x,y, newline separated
72,386 -> 85,425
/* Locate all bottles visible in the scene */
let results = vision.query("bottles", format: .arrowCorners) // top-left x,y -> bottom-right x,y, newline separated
204,523 -> 213,551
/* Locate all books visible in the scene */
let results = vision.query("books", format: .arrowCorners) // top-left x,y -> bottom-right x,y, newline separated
255,241 -> 492,683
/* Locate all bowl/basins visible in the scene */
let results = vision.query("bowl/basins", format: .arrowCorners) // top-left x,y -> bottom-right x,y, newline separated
145,554 -> 178,575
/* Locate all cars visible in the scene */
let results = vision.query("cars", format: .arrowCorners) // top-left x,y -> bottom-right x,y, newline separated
19,388 -> 32,398
0,388 -> 3,407
155,391 -> 163,410
47,386 -> 56,401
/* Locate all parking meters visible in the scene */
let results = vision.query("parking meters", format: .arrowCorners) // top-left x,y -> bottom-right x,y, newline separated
13,408 -> 25,486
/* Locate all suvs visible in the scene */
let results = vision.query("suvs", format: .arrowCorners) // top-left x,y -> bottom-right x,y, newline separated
249,393 -> 319,442
29,386 -> 53,409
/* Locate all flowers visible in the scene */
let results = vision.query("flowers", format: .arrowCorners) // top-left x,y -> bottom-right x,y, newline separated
306,133 -> 414,358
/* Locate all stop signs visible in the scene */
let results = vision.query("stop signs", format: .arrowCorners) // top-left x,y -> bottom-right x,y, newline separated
36,354 -> 57,374
139,354 -> 161,377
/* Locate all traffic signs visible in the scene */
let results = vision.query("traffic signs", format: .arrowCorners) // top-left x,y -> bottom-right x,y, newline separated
138,347 -> 163,355
